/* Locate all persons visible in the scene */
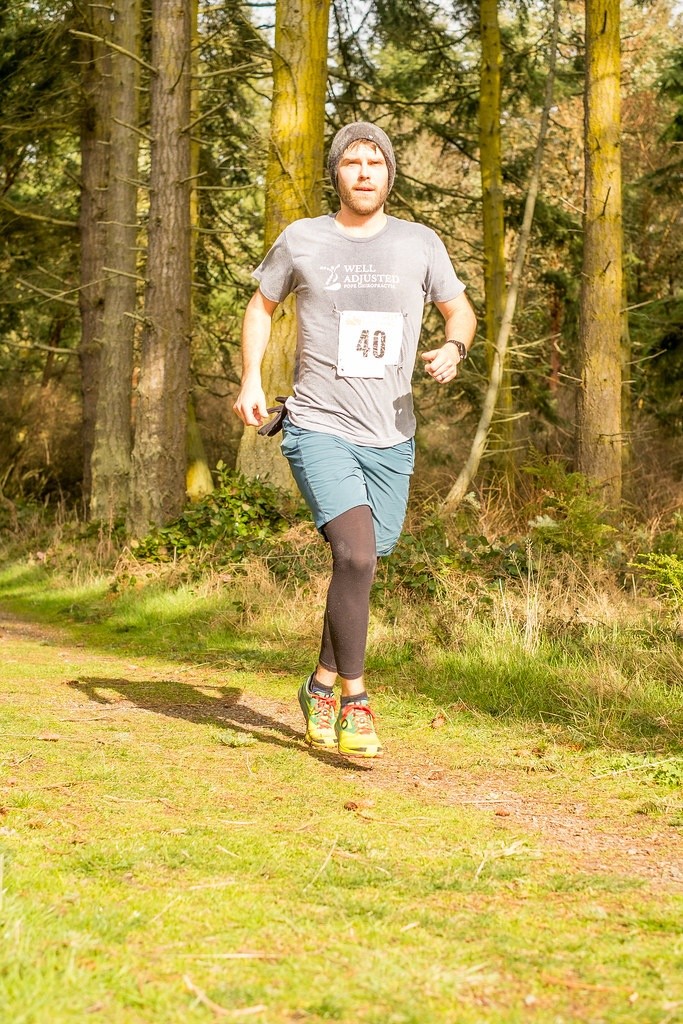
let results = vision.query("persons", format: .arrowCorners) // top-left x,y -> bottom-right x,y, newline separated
232,122 -> 476,758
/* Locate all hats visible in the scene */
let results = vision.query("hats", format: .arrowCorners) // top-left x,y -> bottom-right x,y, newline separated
329,122 -> 396,197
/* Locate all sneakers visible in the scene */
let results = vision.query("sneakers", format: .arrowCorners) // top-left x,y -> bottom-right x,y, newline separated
335,703 -> 384,758
297,667 -> 339,748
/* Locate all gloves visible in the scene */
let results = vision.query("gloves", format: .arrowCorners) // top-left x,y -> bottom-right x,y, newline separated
258,397 -> 288,437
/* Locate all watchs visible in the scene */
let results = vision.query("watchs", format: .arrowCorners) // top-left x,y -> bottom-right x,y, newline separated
447,340 -> 466,359
